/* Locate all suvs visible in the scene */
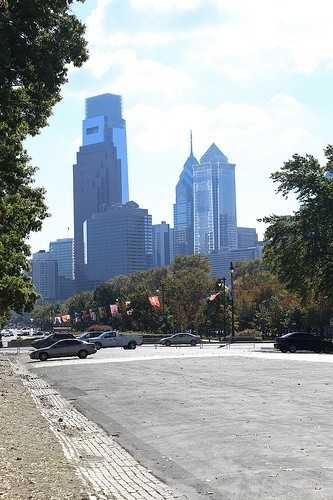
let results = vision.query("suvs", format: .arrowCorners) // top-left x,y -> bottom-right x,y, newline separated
31,332 -> 76,350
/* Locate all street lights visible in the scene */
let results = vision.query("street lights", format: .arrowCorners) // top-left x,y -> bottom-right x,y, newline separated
229,261 -> 238,342
217,277 -> 227,339
155,283 -> 166,334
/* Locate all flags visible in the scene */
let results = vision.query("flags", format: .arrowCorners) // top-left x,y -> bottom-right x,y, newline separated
54,307 -> 108,324
209,293 -> 219,301
109,305 -> 117,316
126,301 -> 133,315
148,296 -> 160,308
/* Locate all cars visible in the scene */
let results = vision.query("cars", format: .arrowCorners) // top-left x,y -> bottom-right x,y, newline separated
17,330 -> 29,336
274,332 -> 333,353
1,329 -> 13,337
29,338 -> 97,361
76,332 -> 104,345
159,333 -> 201,347
32,331 -> 50,336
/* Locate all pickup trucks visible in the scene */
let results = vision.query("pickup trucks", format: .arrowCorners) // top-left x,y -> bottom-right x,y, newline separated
87,331 -> 143,351
